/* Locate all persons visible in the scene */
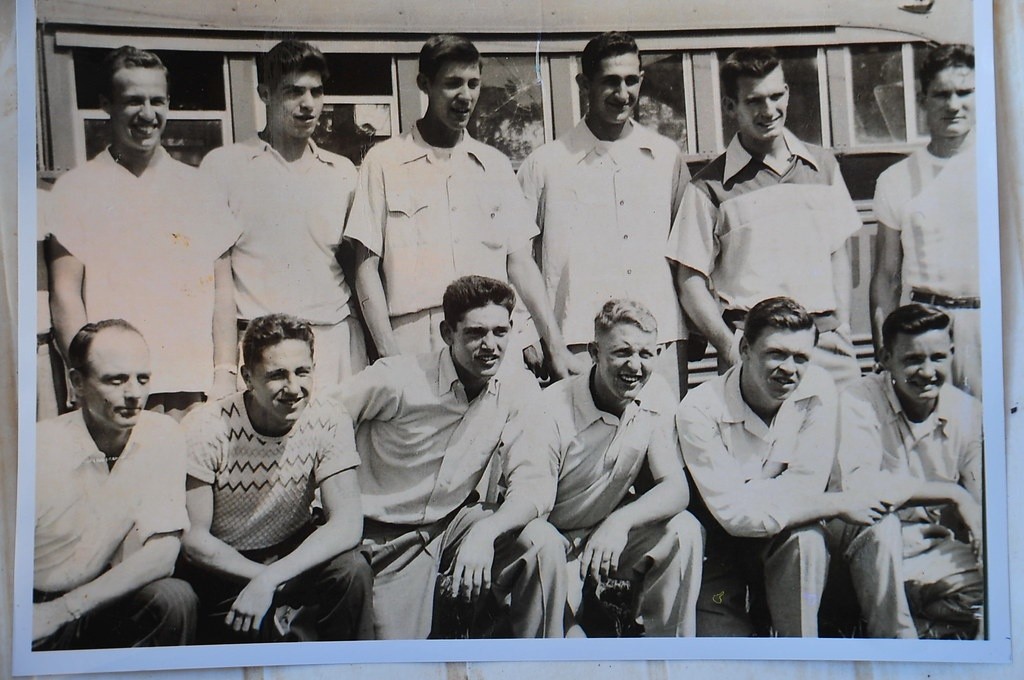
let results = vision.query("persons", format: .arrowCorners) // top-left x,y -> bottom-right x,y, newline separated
340,35 -> 590,378
540,298 -> 705,638
327,276 -> 566,639
194,36 -> 358,399
833,303 -> 987,640
663,47 -> 862,376
40,44 -> 244,425
867,43 -> 984,396
506,30 -> 692,408
33,320 -> 201,652
182,313 -> 373,646
674,296 -> 896,638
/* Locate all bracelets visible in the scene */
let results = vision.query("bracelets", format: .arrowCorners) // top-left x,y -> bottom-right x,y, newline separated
211,363 -> 238,374
62,590 -> 82,621
